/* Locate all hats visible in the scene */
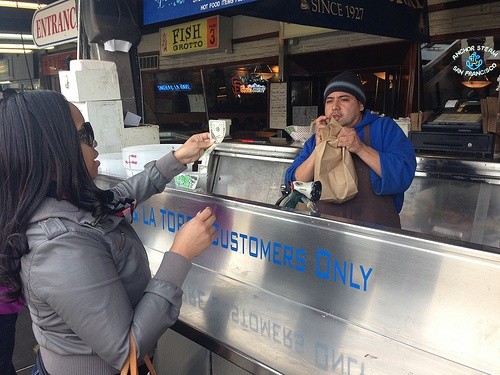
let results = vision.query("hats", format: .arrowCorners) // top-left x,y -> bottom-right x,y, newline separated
322,70 -> 367,110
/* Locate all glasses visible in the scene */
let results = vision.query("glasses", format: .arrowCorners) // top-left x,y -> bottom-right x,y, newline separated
77,122 -> 94,147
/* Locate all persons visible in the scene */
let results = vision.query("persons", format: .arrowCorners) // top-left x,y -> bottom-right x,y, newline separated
284,70 -> 417,230
0,90 -> 218,375
